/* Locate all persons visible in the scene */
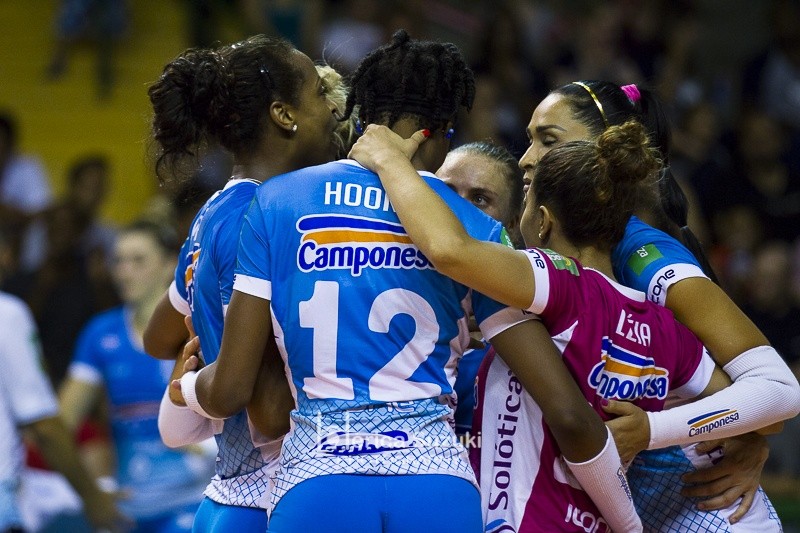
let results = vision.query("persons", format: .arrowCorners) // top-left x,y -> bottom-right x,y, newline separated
0,0 -> 800,533
348,123 -> 785,533
179,30 -> 644,533
